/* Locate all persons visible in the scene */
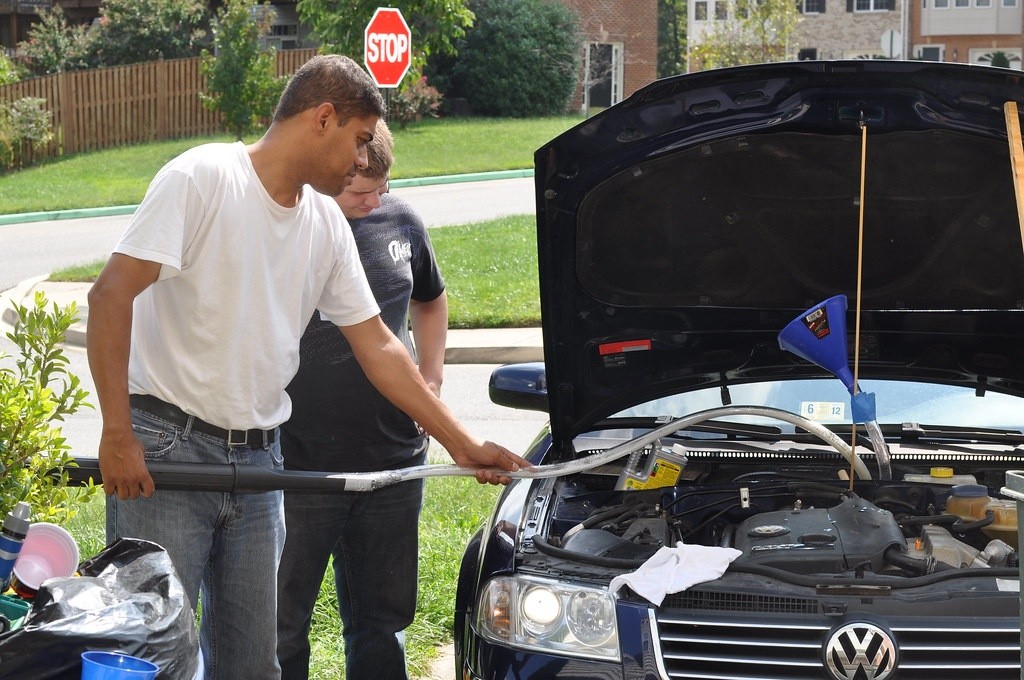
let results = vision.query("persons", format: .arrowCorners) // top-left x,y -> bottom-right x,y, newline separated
88,54 -> 537,680
277,115 -> 447,680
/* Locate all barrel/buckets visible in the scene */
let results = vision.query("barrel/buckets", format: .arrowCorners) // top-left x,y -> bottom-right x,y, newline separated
612,433 -> 689,499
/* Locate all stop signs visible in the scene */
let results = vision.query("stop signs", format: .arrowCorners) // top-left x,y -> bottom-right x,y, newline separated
363,7 -> 412,89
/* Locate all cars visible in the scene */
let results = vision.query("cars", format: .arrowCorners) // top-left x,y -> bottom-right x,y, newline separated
453,59 -> 1024,680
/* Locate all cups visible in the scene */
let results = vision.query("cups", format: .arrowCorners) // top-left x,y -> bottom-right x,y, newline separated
81,651 -> 159,680
9,522 -> 80,598
